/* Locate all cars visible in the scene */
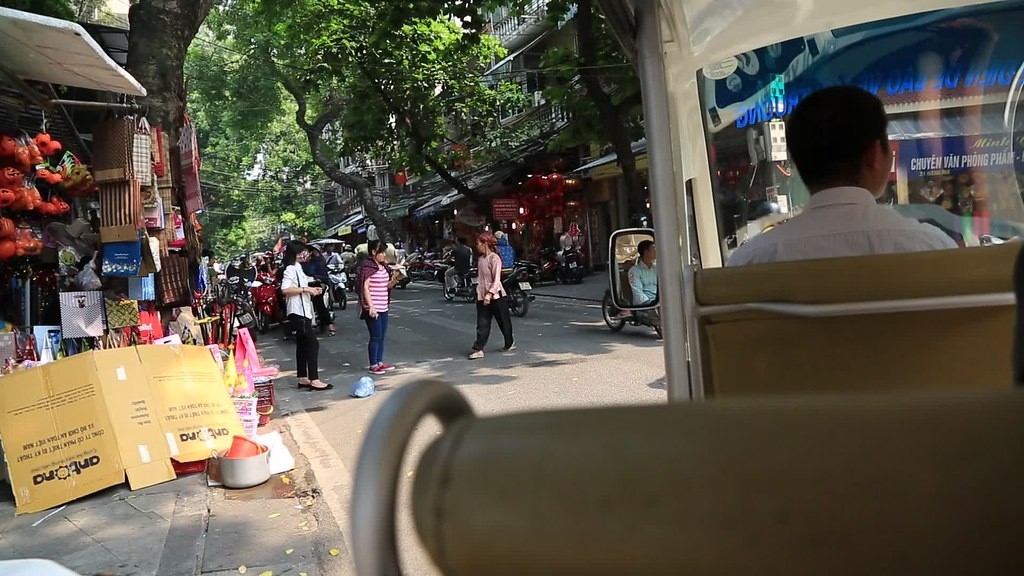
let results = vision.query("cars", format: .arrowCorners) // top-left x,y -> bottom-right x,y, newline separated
718,203 -> 1021,257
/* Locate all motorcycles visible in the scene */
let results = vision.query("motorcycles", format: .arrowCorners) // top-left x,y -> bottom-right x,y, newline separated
602,228 -> 664,337
350,0 -> 1024,576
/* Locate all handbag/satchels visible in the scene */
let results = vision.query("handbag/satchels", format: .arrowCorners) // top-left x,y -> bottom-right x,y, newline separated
300,318 -> 313,334
89,111 -> 188,305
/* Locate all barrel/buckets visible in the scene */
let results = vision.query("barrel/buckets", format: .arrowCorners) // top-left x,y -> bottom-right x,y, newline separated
228,435 -> 266,458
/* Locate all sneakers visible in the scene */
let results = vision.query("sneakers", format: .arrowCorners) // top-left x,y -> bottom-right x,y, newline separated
378,363 -> 395,370
368,365 -> 385,374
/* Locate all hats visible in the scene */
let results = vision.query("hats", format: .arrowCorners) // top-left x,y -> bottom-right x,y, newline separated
495,231 -> 504,239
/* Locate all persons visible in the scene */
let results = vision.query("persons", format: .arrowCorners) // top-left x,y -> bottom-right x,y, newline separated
629,240 -> 662,318
724,85 -> 974,265
202,227 -> 583,390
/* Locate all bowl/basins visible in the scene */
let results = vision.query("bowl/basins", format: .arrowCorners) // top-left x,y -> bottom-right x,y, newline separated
211,445 -> 271,488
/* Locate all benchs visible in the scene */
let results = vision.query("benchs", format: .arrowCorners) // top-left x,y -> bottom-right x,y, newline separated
348,374 -> 1024,575
683,242 -> 1024,396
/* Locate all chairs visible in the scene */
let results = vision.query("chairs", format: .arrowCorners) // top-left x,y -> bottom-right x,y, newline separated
621,261 -> 640,306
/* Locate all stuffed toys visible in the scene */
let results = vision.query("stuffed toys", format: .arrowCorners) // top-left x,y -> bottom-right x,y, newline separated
0,132 -> 101,259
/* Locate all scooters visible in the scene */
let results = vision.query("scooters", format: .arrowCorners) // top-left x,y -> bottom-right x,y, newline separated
214,239 -> 584,318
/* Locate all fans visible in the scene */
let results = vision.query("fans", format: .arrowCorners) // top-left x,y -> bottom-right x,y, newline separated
46,218 -> 100,261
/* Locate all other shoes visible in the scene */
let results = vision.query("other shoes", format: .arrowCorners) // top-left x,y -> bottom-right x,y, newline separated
470,350 -> 484,359
308,382 -> 334,391
329,330 -> 335,337
448,288 -> 456,294
501,341 -> 514,352
298,382 -> 310,389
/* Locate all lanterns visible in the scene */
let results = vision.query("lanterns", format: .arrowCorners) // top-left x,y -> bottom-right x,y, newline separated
518,174 -> 581,210
